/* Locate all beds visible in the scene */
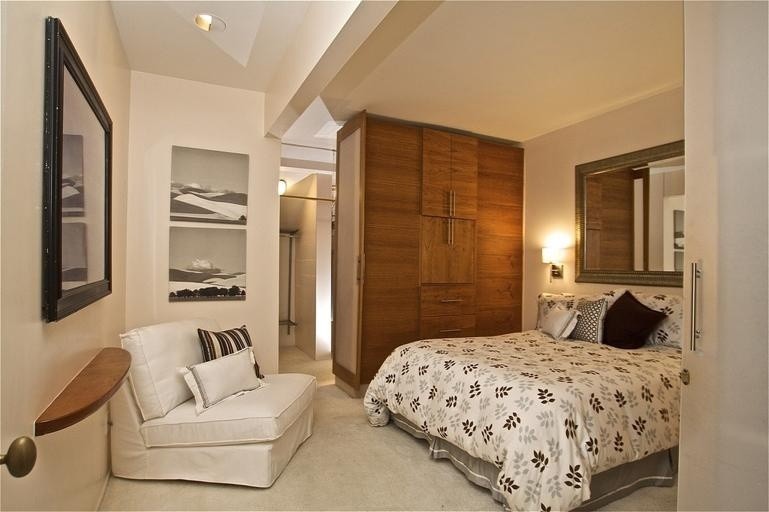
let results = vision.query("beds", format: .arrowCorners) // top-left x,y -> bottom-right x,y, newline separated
359,287 -> 686,511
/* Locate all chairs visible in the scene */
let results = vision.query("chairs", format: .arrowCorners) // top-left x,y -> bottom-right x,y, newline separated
101,320 -> 322,490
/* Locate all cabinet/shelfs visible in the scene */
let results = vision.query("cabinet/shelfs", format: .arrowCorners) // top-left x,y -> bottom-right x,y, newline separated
332,110 -> 526,402
586,168 -> 637,274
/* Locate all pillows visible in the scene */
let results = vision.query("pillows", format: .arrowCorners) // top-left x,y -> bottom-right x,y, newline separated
604,290 -> 685,349
570,298 -> 609,345
535,291 -> 576,333
601,291 -> 668,351
179,345 -> 263,418
538,303 -> 582,342
194,325 -> 264,379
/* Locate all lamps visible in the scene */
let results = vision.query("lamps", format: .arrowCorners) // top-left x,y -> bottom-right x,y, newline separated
541,227 -> 573,283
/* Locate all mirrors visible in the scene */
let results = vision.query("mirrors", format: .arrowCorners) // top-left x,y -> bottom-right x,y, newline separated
573,136 -> 684,289
42,15 -> 116,328
